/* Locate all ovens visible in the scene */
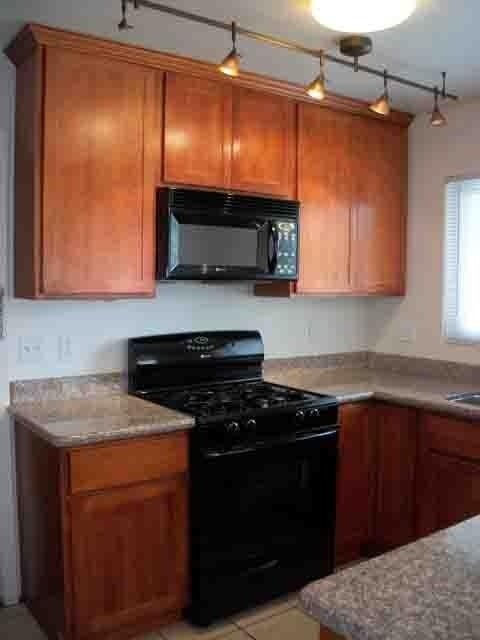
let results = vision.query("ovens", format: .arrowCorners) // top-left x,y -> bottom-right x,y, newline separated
188,431 -> 338,626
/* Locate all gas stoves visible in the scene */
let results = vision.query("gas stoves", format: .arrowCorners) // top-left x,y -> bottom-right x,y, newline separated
132,378 -> 339,443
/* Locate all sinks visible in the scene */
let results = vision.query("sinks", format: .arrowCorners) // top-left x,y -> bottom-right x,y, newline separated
444,389 -> 479,409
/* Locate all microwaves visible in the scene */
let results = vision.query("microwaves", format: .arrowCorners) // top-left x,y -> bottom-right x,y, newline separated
155,188 -> 300,280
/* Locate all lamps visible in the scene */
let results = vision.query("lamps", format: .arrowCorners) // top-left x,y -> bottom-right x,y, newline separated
117,0 -> 459,127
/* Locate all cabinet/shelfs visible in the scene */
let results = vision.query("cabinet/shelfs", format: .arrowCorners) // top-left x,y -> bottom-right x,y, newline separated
162,50 -> 294,199
294,81 -> 406,296
15,400 -> 478,640
15,46 -> 161,300
